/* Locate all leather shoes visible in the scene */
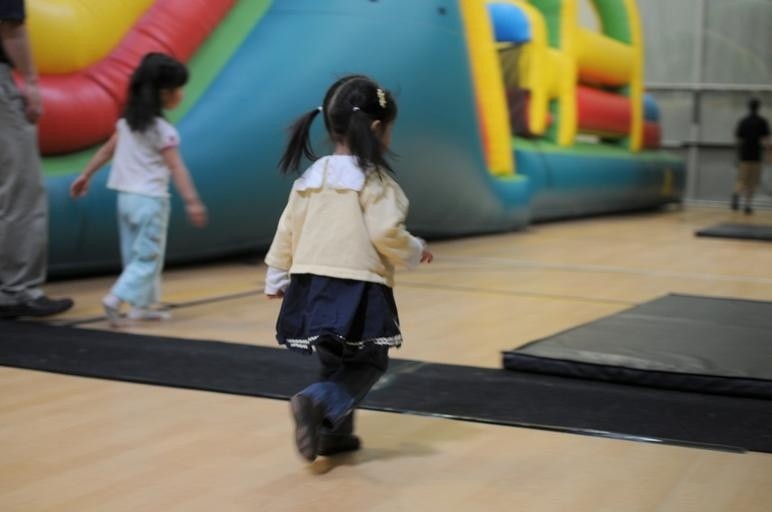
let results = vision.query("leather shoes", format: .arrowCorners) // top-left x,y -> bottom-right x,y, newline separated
1,286 -> 75,320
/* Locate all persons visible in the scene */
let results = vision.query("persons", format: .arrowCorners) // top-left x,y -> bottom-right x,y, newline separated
732,98 -> 772,214
263,75 -> 433,462
70,53 -> 209,329
2,0 -> 73,320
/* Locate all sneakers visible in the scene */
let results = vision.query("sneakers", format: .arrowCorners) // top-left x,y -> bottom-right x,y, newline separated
102,292 -> 171,327
731,194 -> 753,215
290,392 -> 360,461
